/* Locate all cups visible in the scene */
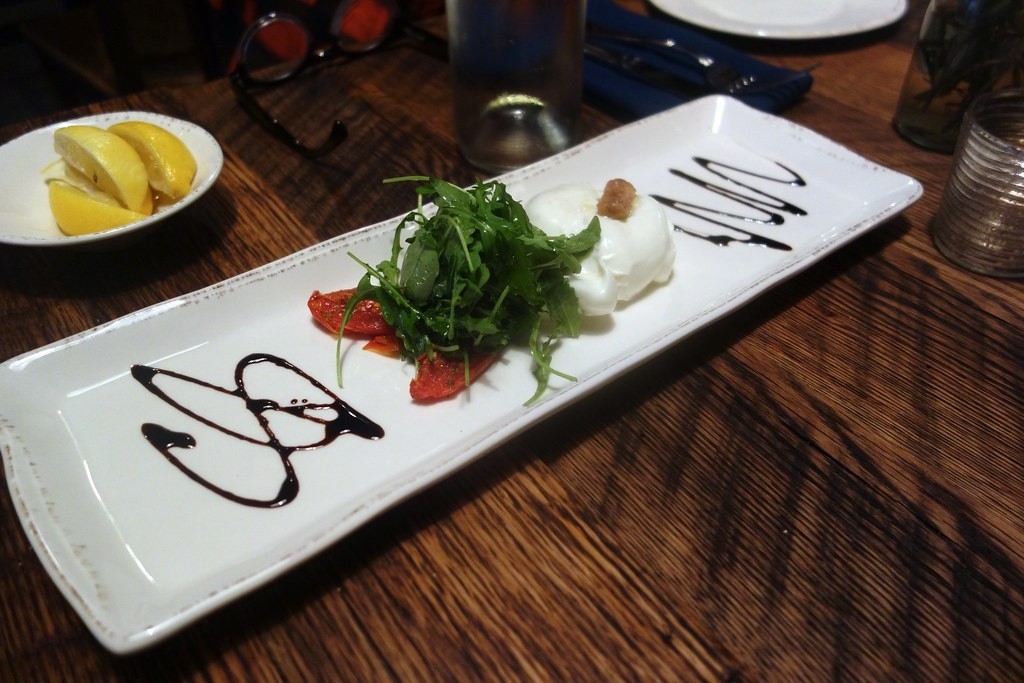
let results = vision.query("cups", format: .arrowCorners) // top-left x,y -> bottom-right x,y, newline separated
445,1 -> 586,174
932,88 -> 1024,278
893,0 -> 1024,152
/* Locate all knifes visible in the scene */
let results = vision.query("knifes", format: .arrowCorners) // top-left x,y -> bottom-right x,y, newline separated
583,42 -> 714,101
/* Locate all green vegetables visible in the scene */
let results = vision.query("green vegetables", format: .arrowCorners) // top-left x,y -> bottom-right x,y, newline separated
334,176 -> 602,407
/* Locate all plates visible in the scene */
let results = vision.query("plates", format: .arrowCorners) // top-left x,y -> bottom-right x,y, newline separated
0,110 -> 224,246
648,1 -> 907,40
0,94 -> 924,656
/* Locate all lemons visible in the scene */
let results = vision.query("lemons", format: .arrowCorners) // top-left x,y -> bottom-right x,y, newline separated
47,121 -> 198,236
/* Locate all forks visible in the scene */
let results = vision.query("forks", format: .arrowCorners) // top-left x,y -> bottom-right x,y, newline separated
585,20 -> 823,95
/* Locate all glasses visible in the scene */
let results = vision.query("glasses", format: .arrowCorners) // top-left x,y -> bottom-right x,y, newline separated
228,0 -> 449,159
